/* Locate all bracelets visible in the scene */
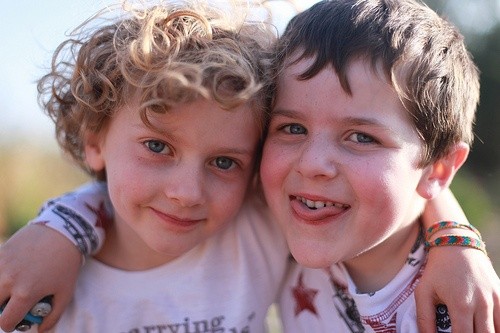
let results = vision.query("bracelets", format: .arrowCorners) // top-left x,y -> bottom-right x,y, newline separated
426,221 -> 481,241
425,235 -> 488,257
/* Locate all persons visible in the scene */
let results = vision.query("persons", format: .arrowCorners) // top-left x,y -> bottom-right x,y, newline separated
30,0 -> 500,333
1,0 -> 451,333
